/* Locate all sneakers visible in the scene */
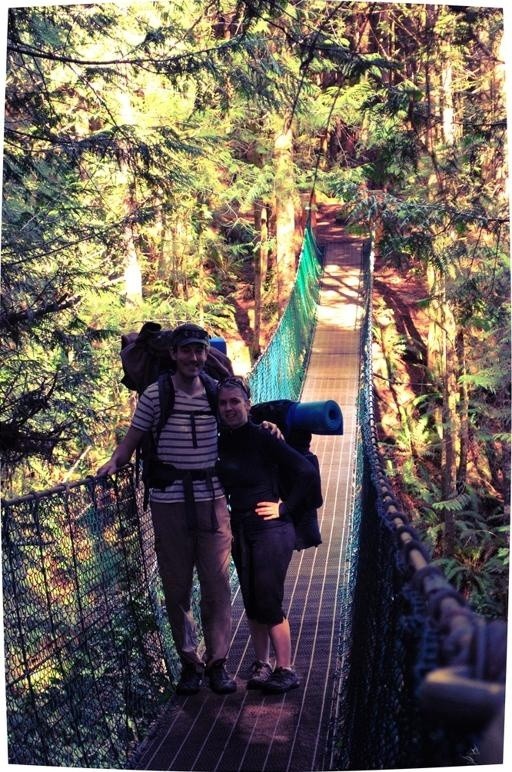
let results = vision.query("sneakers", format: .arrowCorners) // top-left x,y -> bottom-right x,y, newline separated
206,659 -> 237,695
176,668 -> 202,695
246,658 -> 275,689
266,667 -> 300,693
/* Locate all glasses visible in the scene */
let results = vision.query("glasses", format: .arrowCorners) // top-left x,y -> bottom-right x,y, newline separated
218,376 -> 249,397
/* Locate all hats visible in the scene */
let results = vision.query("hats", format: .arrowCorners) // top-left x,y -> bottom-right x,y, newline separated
170,323 -> 209,347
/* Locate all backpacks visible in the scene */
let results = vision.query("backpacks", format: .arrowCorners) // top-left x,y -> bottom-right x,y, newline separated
121,322 -> 217,487
250,400 -> 323,550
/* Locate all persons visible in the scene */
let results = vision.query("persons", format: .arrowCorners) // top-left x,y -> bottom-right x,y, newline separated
216,376 -> 317,694
94,324 -> 284,696
